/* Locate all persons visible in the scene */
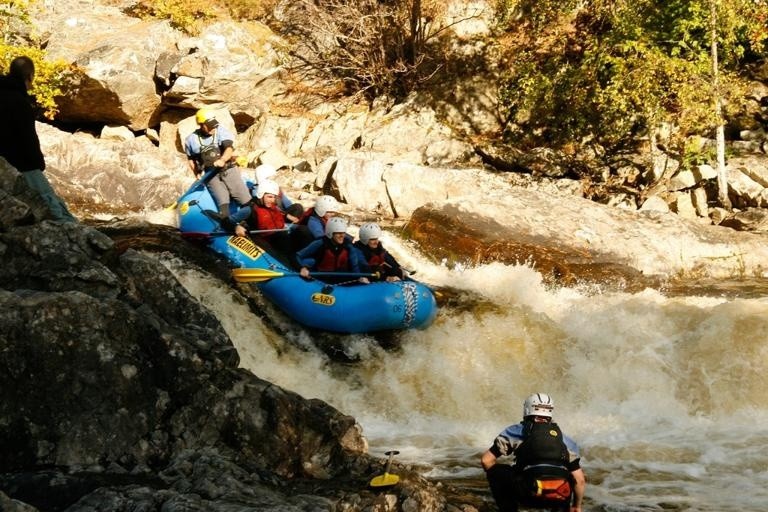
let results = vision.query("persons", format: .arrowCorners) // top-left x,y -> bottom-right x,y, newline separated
185,107 -> 253,219
0,56 -> 78,222
220,164 -> 407,284
481,392 -> 585,512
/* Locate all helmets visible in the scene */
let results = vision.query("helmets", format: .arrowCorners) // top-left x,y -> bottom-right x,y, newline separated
325,217 -> 348,239
314,196 -> 341,217
522,393 -> 555,418
359,223 -> 382,245
256,180 -> 279,199
254,164 -> 278,184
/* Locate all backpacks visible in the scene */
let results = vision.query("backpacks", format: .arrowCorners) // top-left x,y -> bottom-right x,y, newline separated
516,420 -> 571,467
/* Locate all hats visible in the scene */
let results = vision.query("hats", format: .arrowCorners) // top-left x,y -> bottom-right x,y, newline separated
195,108 -> 219,128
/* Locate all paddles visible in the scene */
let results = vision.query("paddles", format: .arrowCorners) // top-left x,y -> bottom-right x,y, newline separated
370,451 -> 400,486
234,268 -> 378,283
180,228 -> 288,239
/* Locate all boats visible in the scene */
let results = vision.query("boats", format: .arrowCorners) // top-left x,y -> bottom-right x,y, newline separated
173,170 -> 439,340
